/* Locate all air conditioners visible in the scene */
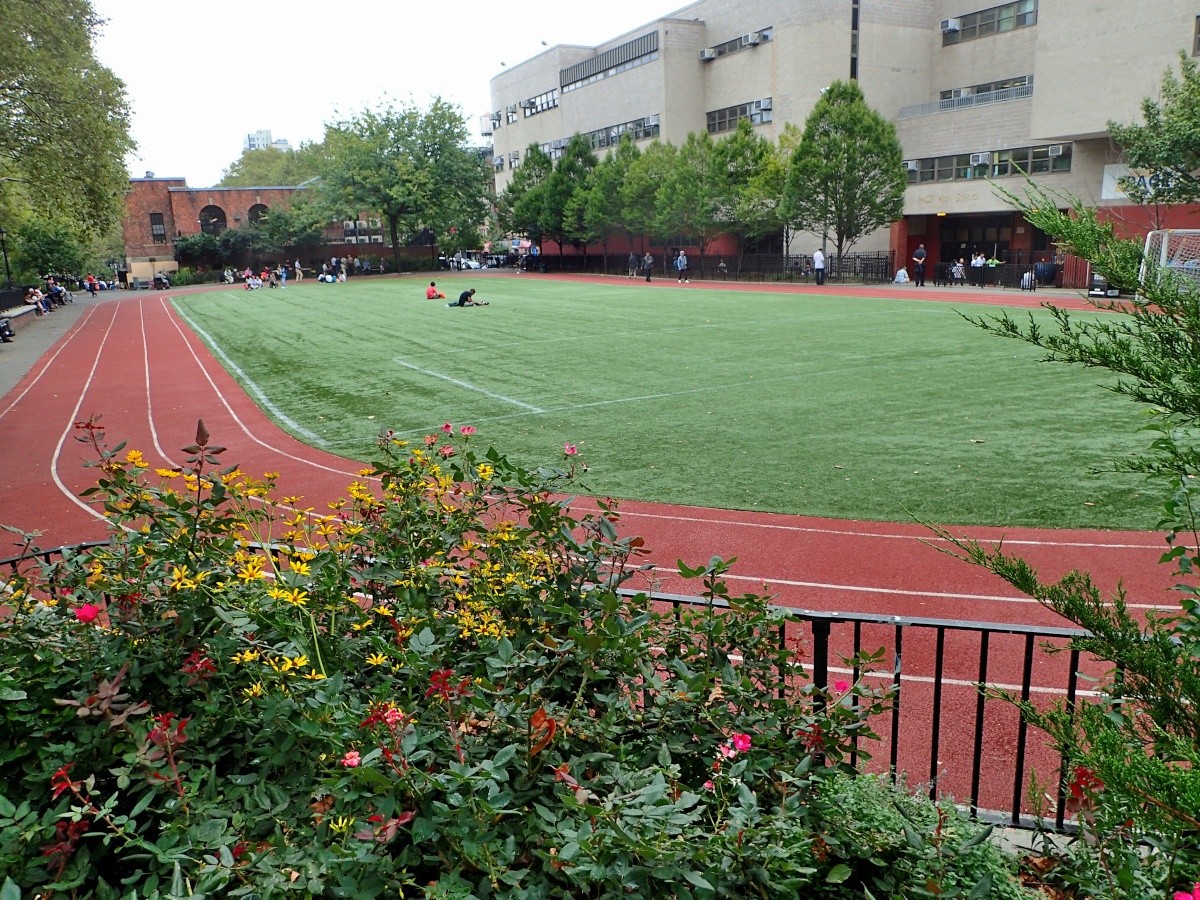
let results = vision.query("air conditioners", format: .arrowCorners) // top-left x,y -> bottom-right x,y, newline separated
742,32 -> 760,46
940,19 -> 960,31
754,99 -> 772,110
1048,146 -> 1065,157
343,219 -> 383,244
900,161 -> 917,171
969,154 -> 989,164
644,115 -> 659,126
952,88 -> 971,99
699,49 -> 715,60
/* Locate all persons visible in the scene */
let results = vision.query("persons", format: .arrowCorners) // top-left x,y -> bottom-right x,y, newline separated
628,251 -> 728,283
0,269 -> 171,344
895,244 -> 1039,292
224,254 -> 388,289
427,251 -> 528,308
794,248 -> 827,285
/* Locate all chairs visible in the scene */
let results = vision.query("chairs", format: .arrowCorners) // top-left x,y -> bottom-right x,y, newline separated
154,278 -> 168,290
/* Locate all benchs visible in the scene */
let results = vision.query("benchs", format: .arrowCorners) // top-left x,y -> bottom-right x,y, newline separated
0,304 -> 38,333
